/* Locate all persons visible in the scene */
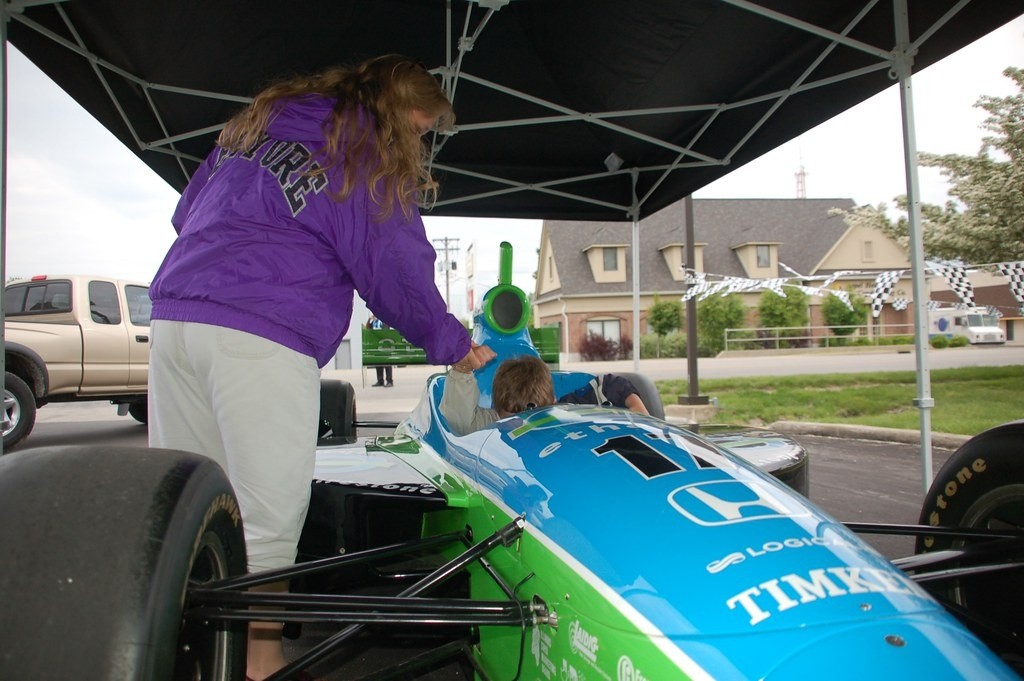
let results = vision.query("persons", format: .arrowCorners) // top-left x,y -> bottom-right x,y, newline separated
438,345 -> 650,438
366,313 -> 394,388
147,51 -> 481,681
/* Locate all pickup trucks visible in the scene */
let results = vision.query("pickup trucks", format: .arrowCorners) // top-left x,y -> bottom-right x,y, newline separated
1,275 -> 157,452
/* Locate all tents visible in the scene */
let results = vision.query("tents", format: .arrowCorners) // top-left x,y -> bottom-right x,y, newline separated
0,0 -> 1024,499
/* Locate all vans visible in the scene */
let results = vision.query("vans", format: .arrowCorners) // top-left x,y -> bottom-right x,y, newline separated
929,307 -> 1007,345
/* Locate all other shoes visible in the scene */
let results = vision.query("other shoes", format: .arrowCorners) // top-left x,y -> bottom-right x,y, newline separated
372,380 -> 384,386
384,380 -> 393,387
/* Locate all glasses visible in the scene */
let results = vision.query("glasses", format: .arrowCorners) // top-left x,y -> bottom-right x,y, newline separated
503,399 -> 538,414
